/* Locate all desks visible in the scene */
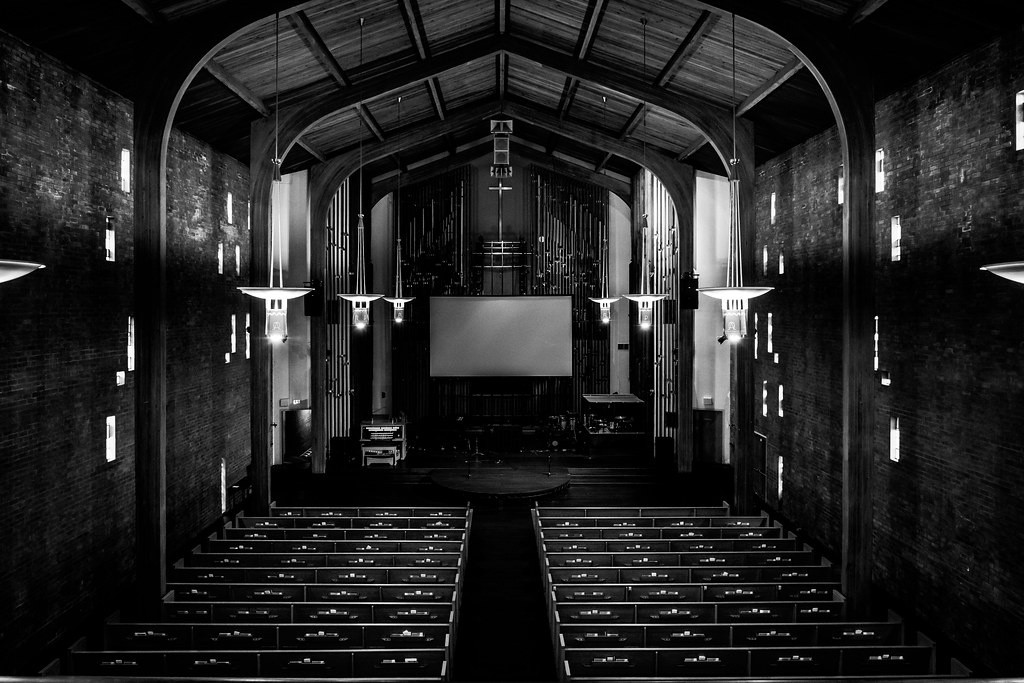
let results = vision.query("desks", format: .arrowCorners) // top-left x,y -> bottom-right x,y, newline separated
360,414 -> 407,464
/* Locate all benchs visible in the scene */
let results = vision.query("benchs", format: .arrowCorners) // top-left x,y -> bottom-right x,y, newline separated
531,504 -> 978,683
35,504 -> 476,682
362,444 -> 398,466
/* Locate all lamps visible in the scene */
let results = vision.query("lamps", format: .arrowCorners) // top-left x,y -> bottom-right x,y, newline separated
588,94 -> 621,323
622,14 -> 672,332
382,96 -> 415,324
234,5 -> 314,350
337,17 -> 384,333
693,9 -> 775,346
0,259 -> 48,286
